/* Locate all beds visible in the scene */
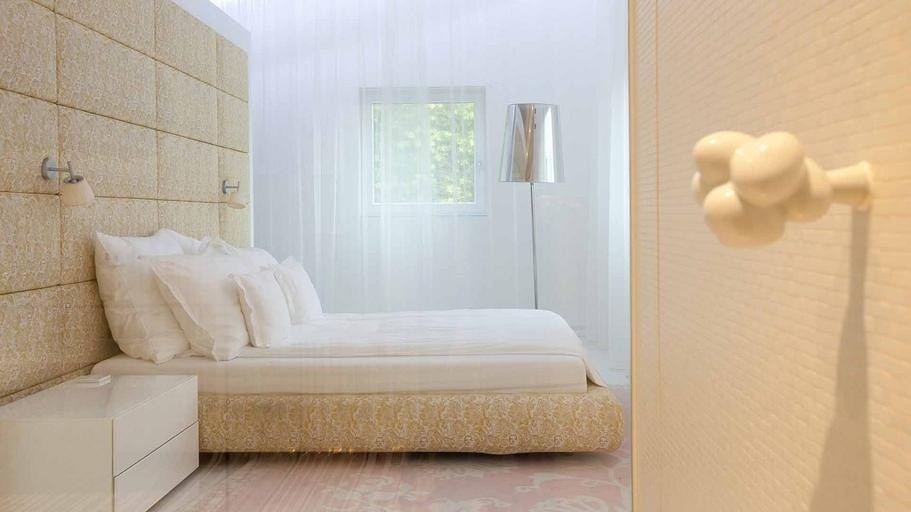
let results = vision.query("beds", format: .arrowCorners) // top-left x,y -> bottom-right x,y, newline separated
89,307 -> 625,456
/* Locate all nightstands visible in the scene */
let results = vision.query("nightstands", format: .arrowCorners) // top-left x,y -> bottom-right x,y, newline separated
1,373 -> 199,512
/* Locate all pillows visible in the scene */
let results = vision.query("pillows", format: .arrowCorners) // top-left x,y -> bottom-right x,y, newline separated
223,266 -> 292,350
274,255 -> 322,325
93,230 -> 196,365
161,227 -> 206,256
137,234 -> 267,368
231,246 -> 279,268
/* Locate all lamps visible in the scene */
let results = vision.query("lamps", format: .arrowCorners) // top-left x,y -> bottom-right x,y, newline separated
41,157 -> 94,207
221,179 -> 245,209
497,101 -> 565,310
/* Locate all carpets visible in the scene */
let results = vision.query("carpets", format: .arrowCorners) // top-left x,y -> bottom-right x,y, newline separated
146,382 -> 633,512
